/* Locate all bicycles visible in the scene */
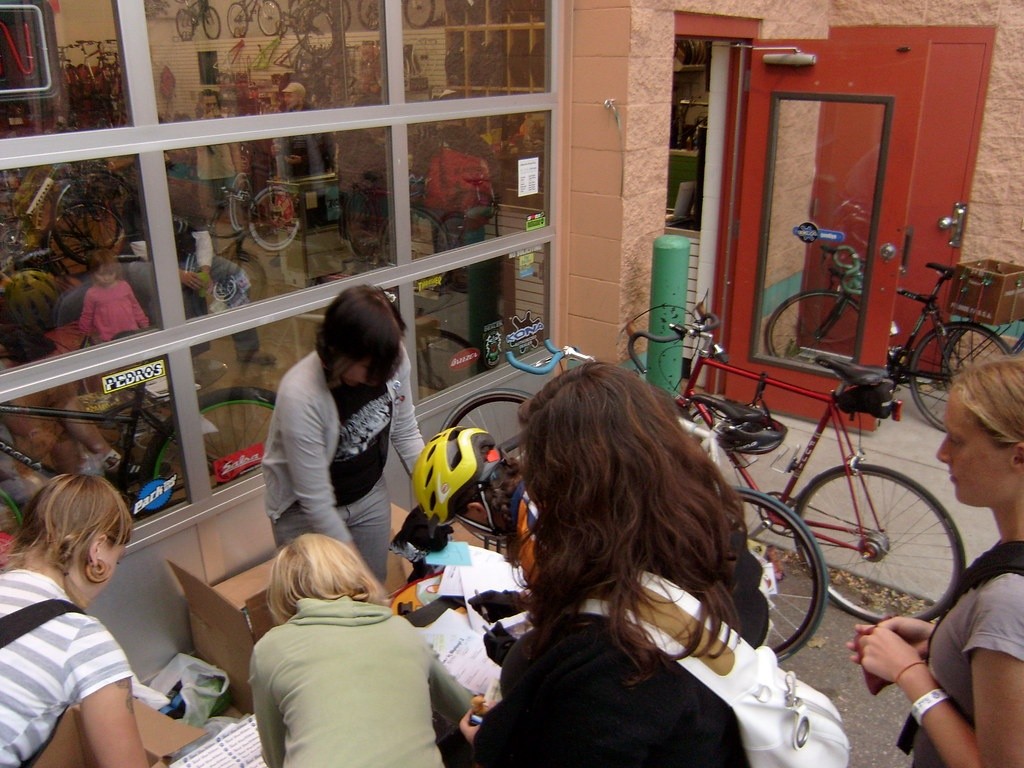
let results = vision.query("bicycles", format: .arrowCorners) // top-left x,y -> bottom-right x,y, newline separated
0,330 -> 279,526
764,245 -> 1024,434
416,319 -> 488,399
0,0 -> 501,318
439,337 -> 826,663
621,288 -> 966,625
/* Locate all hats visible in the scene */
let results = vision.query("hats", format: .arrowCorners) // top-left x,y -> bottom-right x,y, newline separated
281,82 -> 306,97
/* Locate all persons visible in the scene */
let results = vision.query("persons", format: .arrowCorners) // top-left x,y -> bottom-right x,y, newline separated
194,87 -> 246,224
260,283 -> 429,592
843,352 -> 1024,768
280,81 -> 337,234
77,248 -> 160,372
424,124 -> 505,291
412,426 -> 772,651
247,531 -> 476,768
0,470 -> 156,768
458,359 -> 771,768
112,159 -> 279,368
0,272 -> 173,496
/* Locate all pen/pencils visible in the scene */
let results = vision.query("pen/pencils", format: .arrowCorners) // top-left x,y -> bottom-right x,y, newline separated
474,587 -> 490,622
483,625 -> 493,637
469,712 -> 482,722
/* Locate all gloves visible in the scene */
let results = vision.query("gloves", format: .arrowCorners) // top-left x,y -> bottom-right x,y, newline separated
468,588 -> 528,624
483,621 -> 518,666
387,505 -> 453,552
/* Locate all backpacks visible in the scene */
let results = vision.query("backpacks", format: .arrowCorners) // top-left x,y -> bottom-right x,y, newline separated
579,566 -> 853,768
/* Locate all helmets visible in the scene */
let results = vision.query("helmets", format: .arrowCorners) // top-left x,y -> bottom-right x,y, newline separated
411,425 -> 496,540
4,270 -> 59,333
713,408 -> 789,456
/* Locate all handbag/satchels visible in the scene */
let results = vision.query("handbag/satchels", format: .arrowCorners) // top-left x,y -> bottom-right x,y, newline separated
832,378 -> 898,422
440,0 -> 544,99
149,653 -> 234,734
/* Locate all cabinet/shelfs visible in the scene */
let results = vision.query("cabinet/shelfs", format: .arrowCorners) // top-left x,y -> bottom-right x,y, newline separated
443,0 -> 546,143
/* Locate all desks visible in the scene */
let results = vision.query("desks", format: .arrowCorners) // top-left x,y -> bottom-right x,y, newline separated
267,177 -> 346,274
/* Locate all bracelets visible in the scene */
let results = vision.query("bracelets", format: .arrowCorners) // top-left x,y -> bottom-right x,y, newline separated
908,687 -> 951,727
895,657 -> 928,685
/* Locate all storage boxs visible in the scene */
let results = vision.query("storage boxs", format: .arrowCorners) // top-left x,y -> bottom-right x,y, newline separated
948,257 -> 1023,325
28,699 -> 208,768
163,500 -> 411,696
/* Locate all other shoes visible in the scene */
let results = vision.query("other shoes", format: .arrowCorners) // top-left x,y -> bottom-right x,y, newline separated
236,348 -> 277,364
